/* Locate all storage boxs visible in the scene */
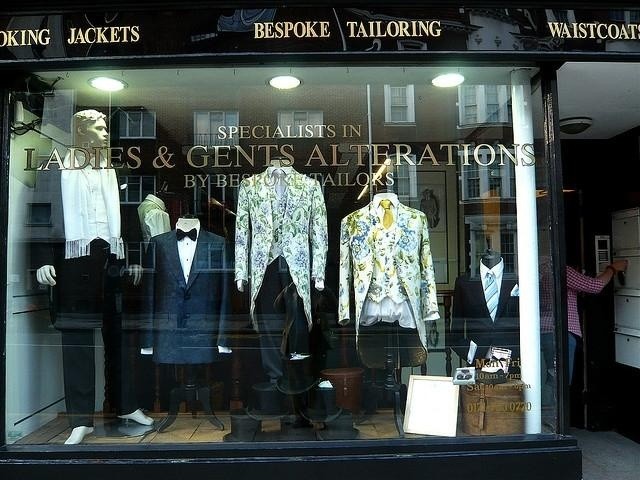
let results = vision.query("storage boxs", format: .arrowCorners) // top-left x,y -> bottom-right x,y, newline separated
461,377 -> 526,437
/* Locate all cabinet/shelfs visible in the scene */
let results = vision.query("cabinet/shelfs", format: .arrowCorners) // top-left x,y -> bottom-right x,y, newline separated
610,209 -> 640,369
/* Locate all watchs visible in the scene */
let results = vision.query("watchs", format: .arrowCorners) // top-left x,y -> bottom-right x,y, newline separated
606,265 -> 617,275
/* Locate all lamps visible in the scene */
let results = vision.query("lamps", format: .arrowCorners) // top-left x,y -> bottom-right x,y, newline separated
555,116 -> 596,137
268,74 -> 303,92
431,73 -> 466,89
86,75 -> 128,94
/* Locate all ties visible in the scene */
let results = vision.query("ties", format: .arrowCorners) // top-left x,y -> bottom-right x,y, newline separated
485,271 -> 499,322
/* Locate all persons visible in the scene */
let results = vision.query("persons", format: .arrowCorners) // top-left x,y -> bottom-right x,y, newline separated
540,235 -> 628,437
30,109 -> 154,444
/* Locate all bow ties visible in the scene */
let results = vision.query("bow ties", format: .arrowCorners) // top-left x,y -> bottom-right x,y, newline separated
176,228 -> 198,240
